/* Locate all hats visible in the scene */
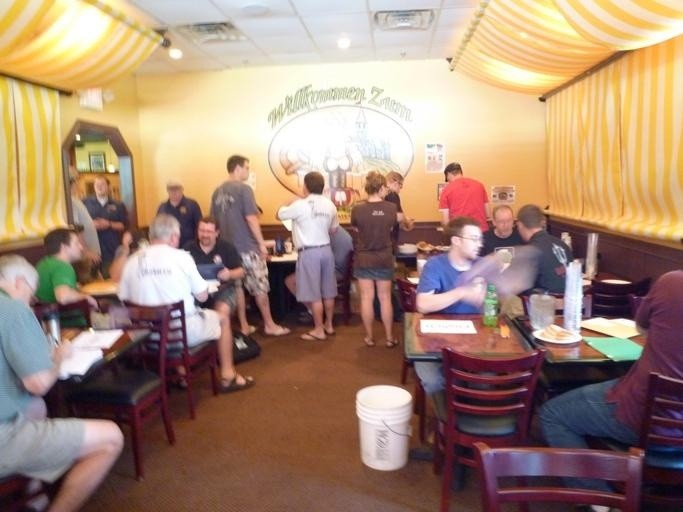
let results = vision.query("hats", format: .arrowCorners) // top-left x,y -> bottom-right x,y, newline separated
445,162 -> 463,182
166,178 -> 181,188
69,166 -> 79,179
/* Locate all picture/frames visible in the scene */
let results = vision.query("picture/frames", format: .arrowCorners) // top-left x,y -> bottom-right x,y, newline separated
86,150 -> 107,174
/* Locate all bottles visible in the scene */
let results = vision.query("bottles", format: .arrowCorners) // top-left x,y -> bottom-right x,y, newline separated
483,284 -> 498,326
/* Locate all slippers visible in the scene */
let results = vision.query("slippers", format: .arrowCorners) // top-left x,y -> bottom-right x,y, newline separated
241,326 -> 256,338
263,325 -> 292,337
324,327 -> 335,336
300,331 -> 327,343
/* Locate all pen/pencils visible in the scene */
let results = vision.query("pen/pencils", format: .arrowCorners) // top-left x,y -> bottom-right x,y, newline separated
50,337 -> 62,349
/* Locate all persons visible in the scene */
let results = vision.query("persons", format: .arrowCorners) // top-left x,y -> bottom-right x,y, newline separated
116,213 -> 255,394
516,205 -> 576,296
69,166 -> 102,280
439,163 -> 491,244
414,216 -> 500,458
155,179 -> 203,249
365,172 -> 415,322
539,270 -> 683,512
182,217 -> 250,317
480,206 -> 527,257
82,176 -> 129,280
351,172 -> 397,349
284,226 -> 354,325
0,254 -> 124,512
32,229 -> 133,330
209,155 -> 290,336
276,172 -> 337,342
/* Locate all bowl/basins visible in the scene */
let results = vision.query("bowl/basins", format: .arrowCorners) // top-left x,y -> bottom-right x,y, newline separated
398,244 -> 417,254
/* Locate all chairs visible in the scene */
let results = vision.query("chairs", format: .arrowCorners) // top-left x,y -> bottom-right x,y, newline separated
396,276 -> 417,313
331,246 -> 355,324
64,301 -> 178,480
125,297 -> 221,423
0,467 -> 46,512
555,294 -> 592,317
638,369 -> 683,512
32,297 -> 94,331
471,441 -> 646,512
590,277 -> 651,314
430,345 -> 548,512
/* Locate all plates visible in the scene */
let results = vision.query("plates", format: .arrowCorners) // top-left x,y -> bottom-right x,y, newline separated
532,329 -> 583,344
435,246 -> 449,251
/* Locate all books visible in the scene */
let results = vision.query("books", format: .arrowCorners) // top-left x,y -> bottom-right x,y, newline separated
55,344 -> 104,379
70,329 -> 122,348
582,337 -> 643,363
420,317 -> 478,335
581,316 -> 639,338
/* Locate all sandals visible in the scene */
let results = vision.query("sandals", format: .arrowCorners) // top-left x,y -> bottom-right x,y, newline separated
211,372 -> 257,396
363,335 -> 375,346
386,338 -> 399,348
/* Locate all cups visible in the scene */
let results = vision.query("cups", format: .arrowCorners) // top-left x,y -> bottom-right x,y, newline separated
529,295 -> 556,330
284,242 -> 292,253
41,312 -> 61,344
275,239 -> 284,253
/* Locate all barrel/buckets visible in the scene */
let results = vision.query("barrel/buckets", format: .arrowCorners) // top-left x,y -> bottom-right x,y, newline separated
355,384 -> 414,471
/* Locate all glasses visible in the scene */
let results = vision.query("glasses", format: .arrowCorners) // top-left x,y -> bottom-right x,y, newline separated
453,234 -> 483,243
24,280 -> 39,307
392,179 -> 404,186
